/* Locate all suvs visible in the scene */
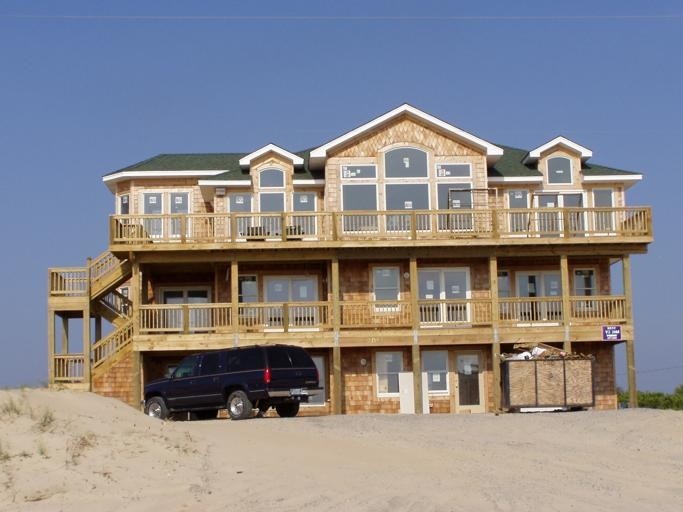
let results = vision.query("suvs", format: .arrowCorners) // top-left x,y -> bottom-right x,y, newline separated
144,343 -> 324,419
